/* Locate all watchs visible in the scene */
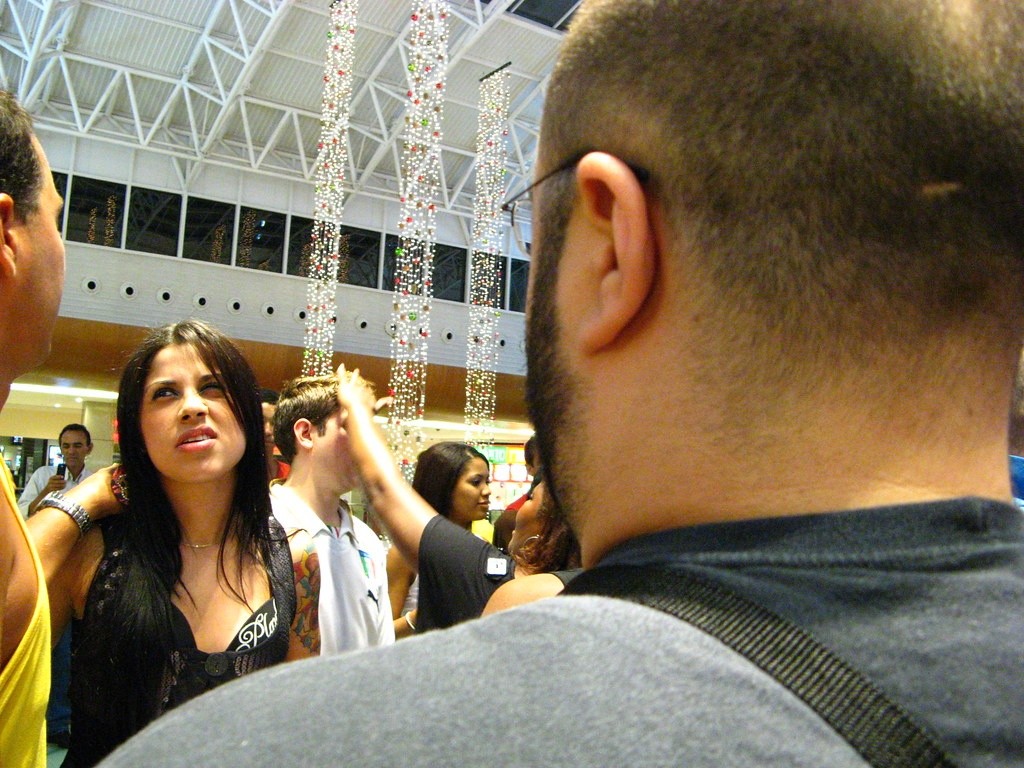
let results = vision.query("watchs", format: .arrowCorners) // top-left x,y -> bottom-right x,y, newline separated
35,491 -> 90,539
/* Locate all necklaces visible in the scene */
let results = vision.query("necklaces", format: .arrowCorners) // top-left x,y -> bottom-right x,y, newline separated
180,538 -> 220,547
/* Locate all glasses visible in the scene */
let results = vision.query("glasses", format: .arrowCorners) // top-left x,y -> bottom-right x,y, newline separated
501,147 -> 650,258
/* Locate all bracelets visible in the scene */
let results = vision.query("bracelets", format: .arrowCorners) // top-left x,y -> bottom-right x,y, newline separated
406,611 -> 415,629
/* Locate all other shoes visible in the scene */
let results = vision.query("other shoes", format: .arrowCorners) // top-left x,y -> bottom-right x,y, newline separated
47,730 -> 71,748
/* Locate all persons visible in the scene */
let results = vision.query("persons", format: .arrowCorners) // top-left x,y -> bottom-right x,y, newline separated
100,0 -> 1024,767
1,88 -> 585,767
18,423 -> 93,748
1010,347 -> 1024,505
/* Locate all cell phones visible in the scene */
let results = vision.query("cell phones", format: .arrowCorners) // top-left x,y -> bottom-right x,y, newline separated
56,463 -> 66,479
